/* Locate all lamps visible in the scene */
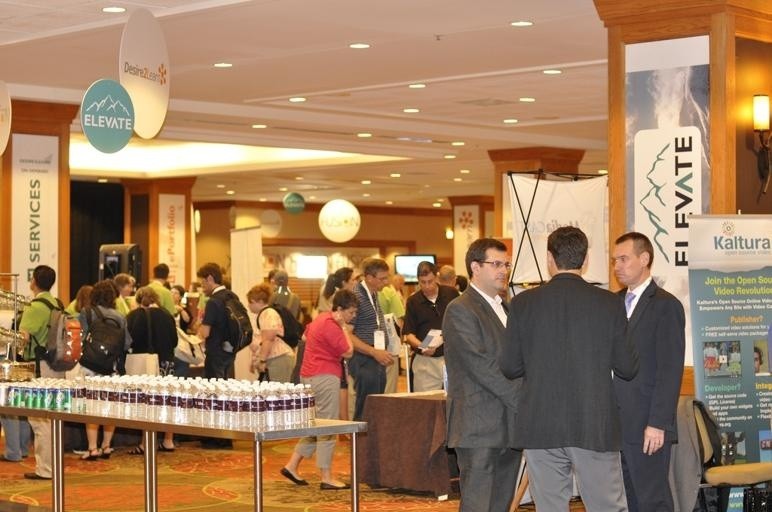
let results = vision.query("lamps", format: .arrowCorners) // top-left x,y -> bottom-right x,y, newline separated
748,93 -> 772,195
317,197 -> 360,244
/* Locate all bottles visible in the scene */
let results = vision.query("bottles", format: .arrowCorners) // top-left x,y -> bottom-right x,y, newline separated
265,386 -> 278,427
287,383 -> 296,425
86,369 -> 196,423
226,388 -> 236,427
0,385 -> 8,407
70,378 -> 83,413
214,385 -> 226,425
196,388 -> 203,424
279,385 -> 291,426
305,385 -> 315,420
192,373 -> 291,391
251,387 -> 264,428
297,384 -> 307,422
204,386 -> 213,426
10,377 -> 76,411
239,386 -> 251,428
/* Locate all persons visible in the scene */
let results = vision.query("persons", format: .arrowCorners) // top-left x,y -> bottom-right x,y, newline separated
402,261 -> 460,393
268,270 -> 278,286
113,274 -> 135,316
347,258 -> 394,423
500,227 -> 639,512
136,263 -> 175,320
18,266 -> 65,480
247,285 -> 297,384
439,264 -> 466,287
0,413 -> 31,462
607,232 -> 686,512
168,286 -> 192,377
75,281 -> 132,461
197,263 -> 236,450
454,275 -> 467,293
272,272 -> 300,321
442,238 -> 524,512
317,268 -> 356,313
125,286 -> 178,455
279,289 -> 360,491
65,285 -> 91,318
377,274 -> 406,394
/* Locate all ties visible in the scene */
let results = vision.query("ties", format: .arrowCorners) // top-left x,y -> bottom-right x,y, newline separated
623,291 -> 637,317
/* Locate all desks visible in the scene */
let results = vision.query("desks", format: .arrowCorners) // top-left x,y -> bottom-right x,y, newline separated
362,391 -> 462,494
0,406 -> 366,512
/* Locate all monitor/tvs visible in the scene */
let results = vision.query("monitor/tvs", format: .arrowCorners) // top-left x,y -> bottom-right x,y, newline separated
394,254 -> 436,285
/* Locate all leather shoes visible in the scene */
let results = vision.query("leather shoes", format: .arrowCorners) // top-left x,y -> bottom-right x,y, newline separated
200,437 -> 233,450
1,454 -> 11,461
281,468 -> 309,485
320,481 -> 351,490
24,472 -> 50,480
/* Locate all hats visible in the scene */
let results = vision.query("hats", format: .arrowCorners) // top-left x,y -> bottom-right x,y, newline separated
275,270 -> 289,281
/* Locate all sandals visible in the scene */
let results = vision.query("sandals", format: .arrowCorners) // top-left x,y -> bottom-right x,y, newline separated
98,447 -> 111,458
80,448 -> 99,461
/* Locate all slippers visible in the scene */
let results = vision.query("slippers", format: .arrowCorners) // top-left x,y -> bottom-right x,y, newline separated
157,443 -> 175,451
128,446 -> 145,454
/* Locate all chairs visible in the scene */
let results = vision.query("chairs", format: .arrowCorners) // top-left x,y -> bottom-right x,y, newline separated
693,395 -> 772,512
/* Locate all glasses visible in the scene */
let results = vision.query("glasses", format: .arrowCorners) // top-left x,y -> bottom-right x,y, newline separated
610,254 -> 640,264
480,261 -> 511,269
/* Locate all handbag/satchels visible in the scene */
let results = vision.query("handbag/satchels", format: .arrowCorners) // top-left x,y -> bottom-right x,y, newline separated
175,327 -> 205,364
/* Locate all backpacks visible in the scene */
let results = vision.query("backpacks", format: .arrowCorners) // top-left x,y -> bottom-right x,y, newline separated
26,298 -> 82,371
78,305 -> 125,377
211,289 -> 252,355
